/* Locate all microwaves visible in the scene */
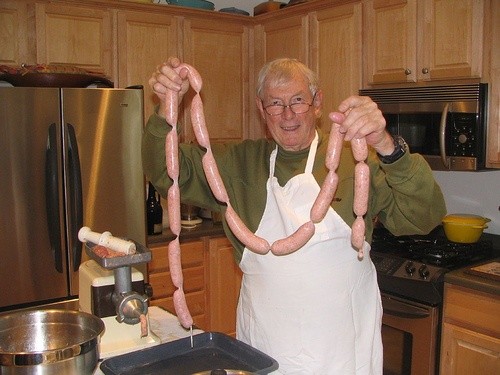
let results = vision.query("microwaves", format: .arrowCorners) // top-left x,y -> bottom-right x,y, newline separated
357,82 -> 499,172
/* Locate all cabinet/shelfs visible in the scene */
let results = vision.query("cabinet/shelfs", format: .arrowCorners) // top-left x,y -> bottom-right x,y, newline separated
253,0 -> 500,172
439,280 -> 500,375
149,237 -> 248,339
0,0 -> 253,154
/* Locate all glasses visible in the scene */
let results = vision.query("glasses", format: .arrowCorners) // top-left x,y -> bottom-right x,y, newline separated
261,88 -> 319,116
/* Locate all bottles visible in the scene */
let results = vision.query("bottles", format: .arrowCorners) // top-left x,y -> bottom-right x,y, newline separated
146,179 -> 162,236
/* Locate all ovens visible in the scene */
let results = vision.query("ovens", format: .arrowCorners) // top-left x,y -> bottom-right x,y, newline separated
371,273 -> 441,375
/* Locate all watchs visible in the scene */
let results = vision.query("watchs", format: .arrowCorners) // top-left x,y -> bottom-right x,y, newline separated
376,135 -> 408,164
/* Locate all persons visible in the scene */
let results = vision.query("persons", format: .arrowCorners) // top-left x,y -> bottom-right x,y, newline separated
141,56 -> 447,375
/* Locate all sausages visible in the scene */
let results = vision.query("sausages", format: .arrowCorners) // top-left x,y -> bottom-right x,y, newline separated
166,63 -> 369,329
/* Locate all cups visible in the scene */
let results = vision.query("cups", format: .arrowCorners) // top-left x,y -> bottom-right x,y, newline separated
211,210 -> 223,225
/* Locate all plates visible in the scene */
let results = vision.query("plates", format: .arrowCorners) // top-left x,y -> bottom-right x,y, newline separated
132,0 -> 250,16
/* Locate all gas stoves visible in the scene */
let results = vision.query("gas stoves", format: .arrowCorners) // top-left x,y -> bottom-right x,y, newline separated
364,215 -> 500,306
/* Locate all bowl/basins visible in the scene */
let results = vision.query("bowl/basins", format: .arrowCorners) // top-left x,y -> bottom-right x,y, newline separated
0,309 -> 106,375
442,213 -> 492,243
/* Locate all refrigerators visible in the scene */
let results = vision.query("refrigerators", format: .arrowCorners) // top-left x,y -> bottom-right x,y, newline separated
0,80 -> 150,324
